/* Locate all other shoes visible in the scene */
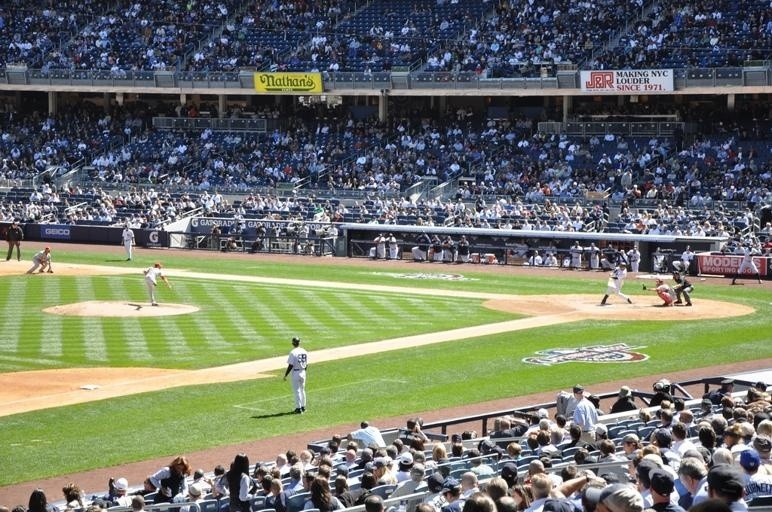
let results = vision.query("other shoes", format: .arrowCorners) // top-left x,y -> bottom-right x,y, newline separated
294,408 -> 302,414
301,407 -> 305,412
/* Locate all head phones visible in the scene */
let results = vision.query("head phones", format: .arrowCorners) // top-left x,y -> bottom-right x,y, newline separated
654,386 -> 664,392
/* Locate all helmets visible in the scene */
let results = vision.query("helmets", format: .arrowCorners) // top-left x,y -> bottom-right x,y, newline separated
292,337 -> 300,343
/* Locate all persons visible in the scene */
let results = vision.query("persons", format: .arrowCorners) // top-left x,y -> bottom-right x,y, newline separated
598,262 -> 635,305
141,262 -> 175,306
672,270 -> 696,306
281,336 -> 310,416
0,379 -> 772,511
641,276 -> 675,307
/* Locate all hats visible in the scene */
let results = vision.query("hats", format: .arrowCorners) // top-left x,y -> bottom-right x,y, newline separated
523,379 -> 772,512
94,418 -> 522,512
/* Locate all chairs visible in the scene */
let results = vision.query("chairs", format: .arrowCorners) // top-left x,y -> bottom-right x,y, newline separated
0,120 -> 746,235
2,2 -> 749,79
66,401 -> 771,510
